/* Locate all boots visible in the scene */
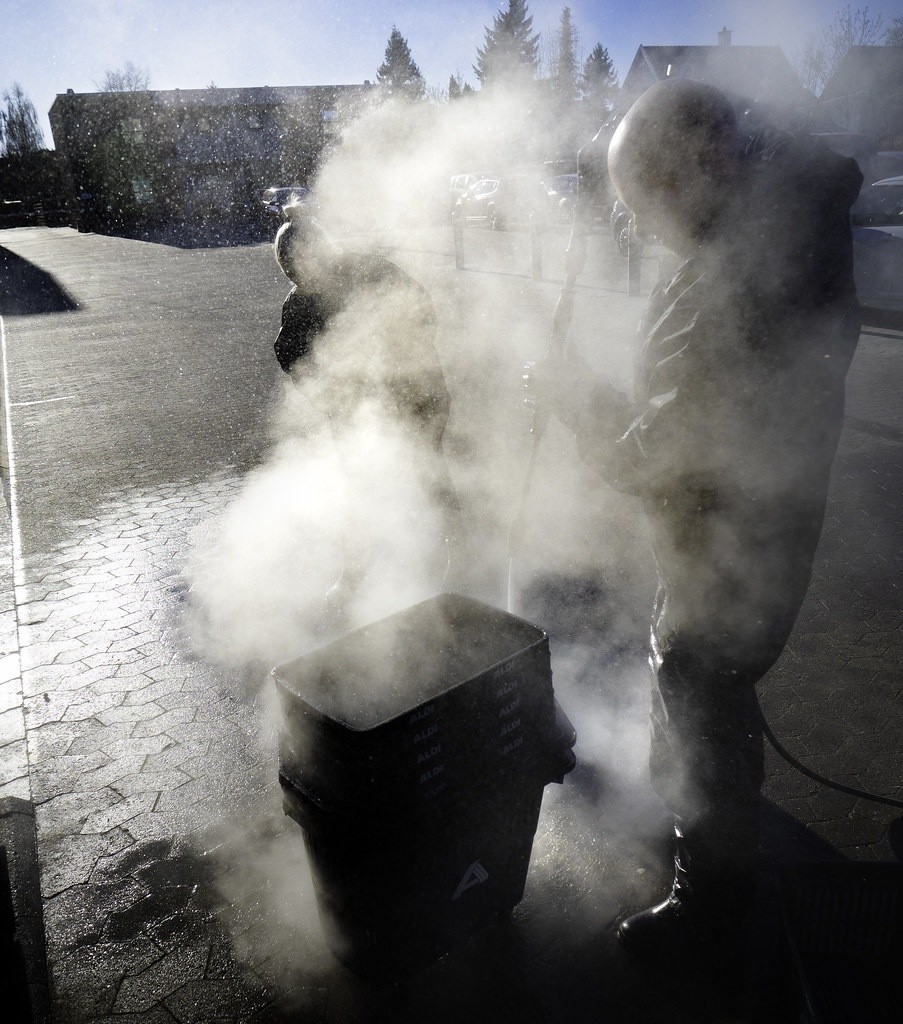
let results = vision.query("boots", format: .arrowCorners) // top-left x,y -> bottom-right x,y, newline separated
616,835 -> 767,955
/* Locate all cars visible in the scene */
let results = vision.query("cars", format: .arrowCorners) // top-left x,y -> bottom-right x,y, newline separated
452,160 -> 630,256
262,187 -> 311,218
849,150 -> 903,311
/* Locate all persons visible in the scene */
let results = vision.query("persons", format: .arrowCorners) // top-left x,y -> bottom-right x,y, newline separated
539,76 -> 863,954
271,198 -> 469,618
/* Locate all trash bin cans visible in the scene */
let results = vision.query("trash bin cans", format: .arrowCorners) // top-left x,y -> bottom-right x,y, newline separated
268,587 -> 579,974
